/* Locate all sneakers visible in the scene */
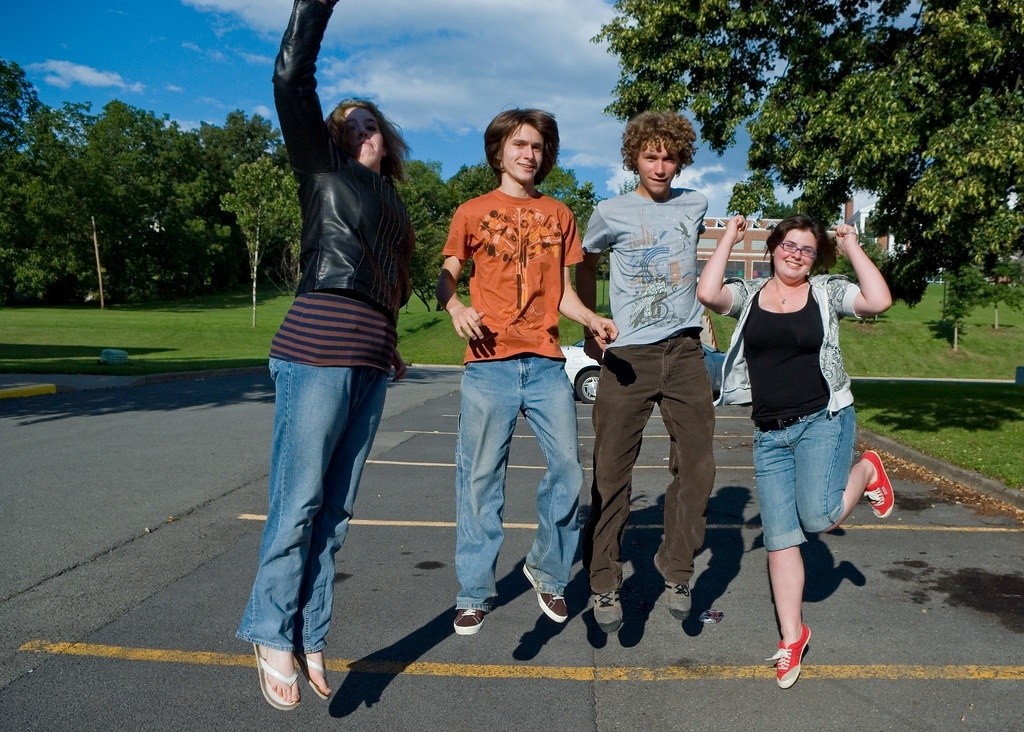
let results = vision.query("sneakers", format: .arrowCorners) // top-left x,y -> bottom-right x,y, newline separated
593,589 -> 623,633
861,450 -> 894,519
653,556 -> 692,623
454,609 -> 484,635
523,563 -> 568,623
765,624 -> 812,688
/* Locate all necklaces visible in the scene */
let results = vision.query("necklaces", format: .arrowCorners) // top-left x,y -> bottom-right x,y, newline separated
781,299 -> 785,303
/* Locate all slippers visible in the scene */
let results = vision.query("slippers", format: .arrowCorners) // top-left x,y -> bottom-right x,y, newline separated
292,647 -> 331,702
253,641 -> 302,711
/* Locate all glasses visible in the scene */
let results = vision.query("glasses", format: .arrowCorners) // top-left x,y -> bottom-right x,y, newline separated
780,241 -> 817,259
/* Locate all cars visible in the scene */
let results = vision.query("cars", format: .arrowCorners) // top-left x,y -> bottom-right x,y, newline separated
558,331 -> 731,405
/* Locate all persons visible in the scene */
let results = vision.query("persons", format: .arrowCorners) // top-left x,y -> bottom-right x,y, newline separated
698,214 -> 894,688
574,111 -> 715,632
234,0 -> 416,709
436,109 -> 619,636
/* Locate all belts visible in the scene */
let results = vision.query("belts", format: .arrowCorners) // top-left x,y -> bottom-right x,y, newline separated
767,418 -> 798,428
